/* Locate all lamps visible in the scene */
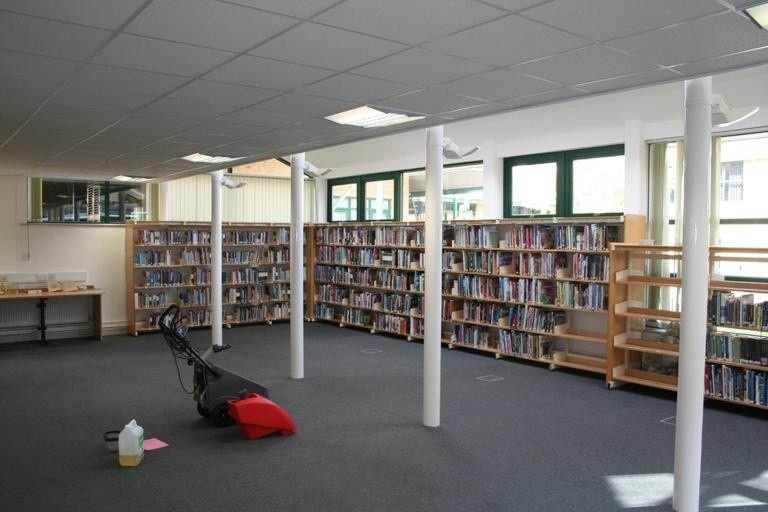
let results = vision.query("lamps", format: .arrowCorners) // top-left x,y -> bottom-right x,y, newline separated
222,177 -> 247,189
443,137 -> 480,159
304,161 -> 335,178
712,94 -> 760,128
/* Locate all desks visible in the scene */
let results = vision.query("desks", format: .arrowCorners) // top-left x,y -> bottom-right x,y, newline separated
0,285 -> 105,346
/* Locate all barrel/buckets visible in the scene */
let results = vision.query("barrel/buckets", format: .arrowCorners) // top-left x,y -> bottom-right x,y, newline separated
118,418 -> 144,468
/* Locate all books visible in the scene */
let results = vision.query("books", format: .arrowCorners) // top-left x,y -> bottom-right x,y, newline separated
134,230 -> 213,328
222,229 -> 291,321
564,226 -> 618,311
442,226 -> 568,360
639,289 -> 767,409
302,228 -> 425,337
